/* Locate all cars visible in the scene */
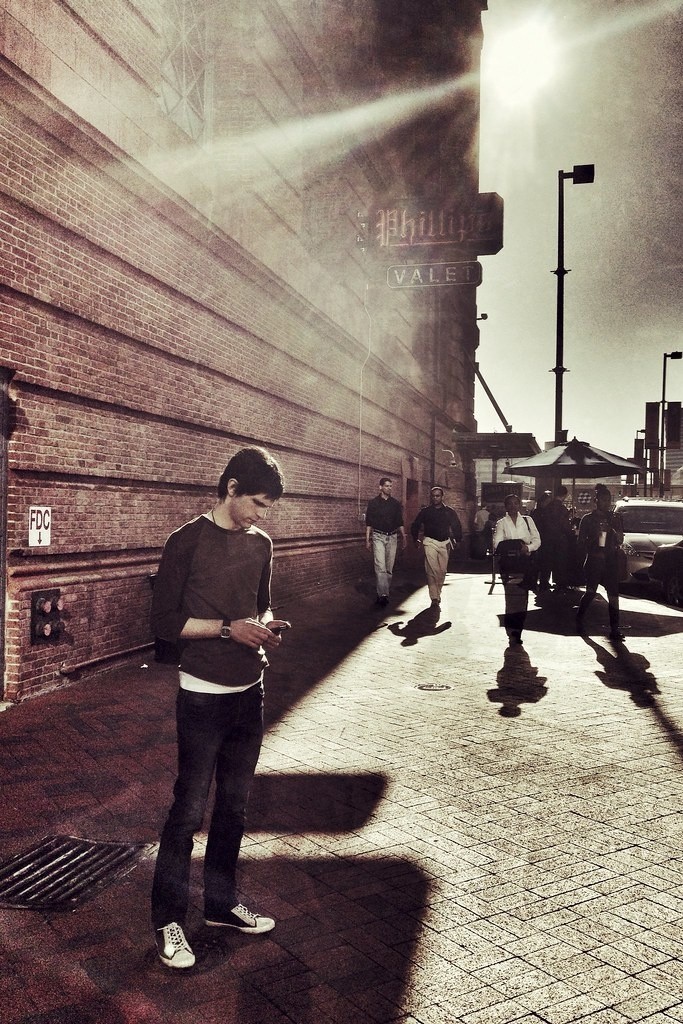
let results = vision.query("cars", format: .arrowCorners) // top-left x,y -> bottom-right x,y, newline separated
611,496 -> 683,593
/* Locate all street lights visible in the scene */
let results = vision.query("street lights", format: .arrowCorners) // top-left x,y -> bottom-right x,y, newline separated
548,164 -> 595,447
659,351 -> 682,498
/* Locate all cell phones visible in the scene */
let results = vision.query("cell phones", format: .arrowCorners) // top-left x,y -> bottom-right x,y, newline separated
268,625 -> 289,632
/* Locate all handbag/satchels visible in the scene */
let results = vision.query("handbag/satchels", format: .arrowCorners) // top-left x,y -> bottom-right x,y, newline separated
612,547 -> 628,583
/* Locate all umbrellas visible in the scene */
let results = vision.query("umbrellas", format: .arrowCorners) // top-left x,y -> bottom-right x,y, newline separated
502,436 -> 647,517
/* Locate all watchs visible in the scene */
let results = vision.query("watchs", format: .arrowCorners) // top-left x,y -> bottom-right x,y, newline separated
221,620 -> 231,638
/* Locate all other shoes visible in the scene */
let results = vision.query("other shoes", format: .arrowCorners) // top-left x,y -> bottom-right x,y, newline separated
576,617 -> 586,636
378,595 -> 390,608
609,630 -> 624,640
430,602 -> 442,612
509,637 -> 523,652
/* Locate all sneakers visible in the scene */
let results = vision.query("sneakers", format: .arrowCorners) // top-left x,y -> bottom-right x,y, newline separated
203,901 -> 274,933
154,922 -> 194,967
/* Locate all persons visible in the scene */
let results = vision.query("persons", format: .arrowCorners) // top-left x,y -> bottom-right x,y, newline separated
150,446 -> 291,968
532,484 -> 572,589
366,477 -> 407,605
576,483 -> 625,640
474,503 -> 490,558
410,487 -> 462,612
493,494 -> 541,644
